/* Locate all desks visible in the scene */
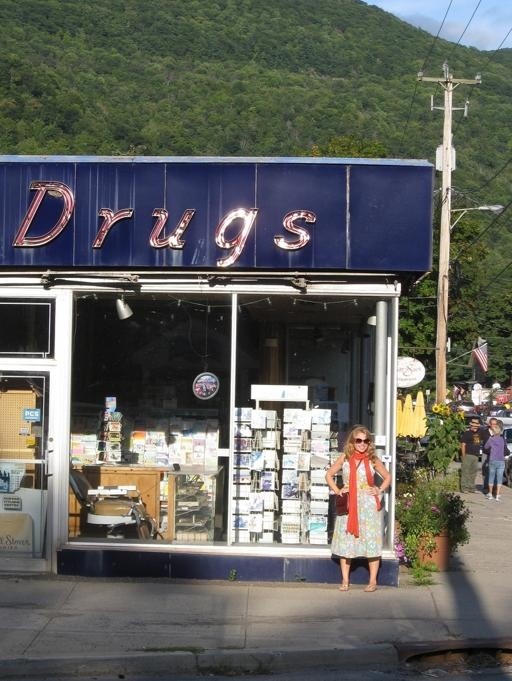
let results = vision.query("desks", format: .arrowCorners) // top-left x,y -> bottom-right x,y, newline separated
69,464 -> 223,542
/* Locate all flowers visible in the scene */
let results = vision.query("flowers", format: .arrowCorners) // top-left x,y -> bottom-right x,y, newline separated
395,481 -> 471,582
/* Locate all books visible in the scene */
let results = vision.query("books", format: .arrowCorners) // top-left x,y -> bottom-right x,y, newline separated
70,416 -> 220,523
232,407 -> 331,547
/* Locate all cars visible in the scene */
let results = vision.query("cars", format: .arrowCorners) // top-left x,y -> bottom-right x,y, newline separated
444,401 -> 512,487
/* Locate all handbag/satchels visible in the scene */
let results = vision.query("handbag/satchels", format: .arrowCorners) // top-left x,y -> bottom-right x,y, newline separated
336,492 -> 349,515
504,445 -> 510,456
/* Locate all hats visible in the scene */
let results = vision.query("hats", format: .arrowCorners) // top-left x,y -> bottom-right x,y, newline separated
469,420 -> 481,426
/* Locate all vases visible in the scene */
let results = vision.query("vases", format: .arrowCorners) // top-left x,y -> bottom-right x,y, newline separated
416,526 -> 449,572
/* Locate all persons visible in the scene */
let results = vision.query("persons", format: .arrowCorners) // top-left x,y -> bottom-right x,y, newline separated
325,426 -> 392,592
461,417 -> 505,501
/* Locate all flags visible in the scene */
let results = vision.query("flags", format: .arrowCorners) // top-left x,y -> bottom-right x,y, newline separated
473,344 -> 488,372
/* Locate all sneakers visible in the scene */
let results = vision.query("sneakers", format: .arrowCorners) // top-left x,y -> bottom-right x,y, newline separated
464,489 -> 500,501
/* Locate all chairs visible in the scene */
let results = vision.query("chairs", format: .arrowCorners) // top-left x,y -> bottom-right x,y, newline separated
69,469 -> 149,538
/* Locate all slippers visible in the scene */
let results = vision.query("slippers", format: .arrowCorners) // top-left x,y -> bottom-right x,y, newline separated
339,585 -> 377,591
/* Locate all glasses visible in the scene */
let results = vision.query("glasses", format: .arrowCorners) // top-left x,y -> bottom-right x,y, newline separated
356,439 -> 370,444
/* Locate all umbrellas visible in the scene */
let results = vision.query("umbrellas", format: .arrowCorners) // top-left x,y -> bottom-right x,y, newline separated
396,390 -> 428,454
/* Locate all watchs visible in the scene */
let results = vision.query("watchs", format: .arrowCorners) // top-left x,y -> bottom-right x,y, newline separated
378,486 -> 384,493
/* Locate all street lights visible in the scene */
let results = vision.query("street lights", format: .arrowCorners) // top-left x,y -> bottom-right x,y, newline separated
435,203 -> 506,412
